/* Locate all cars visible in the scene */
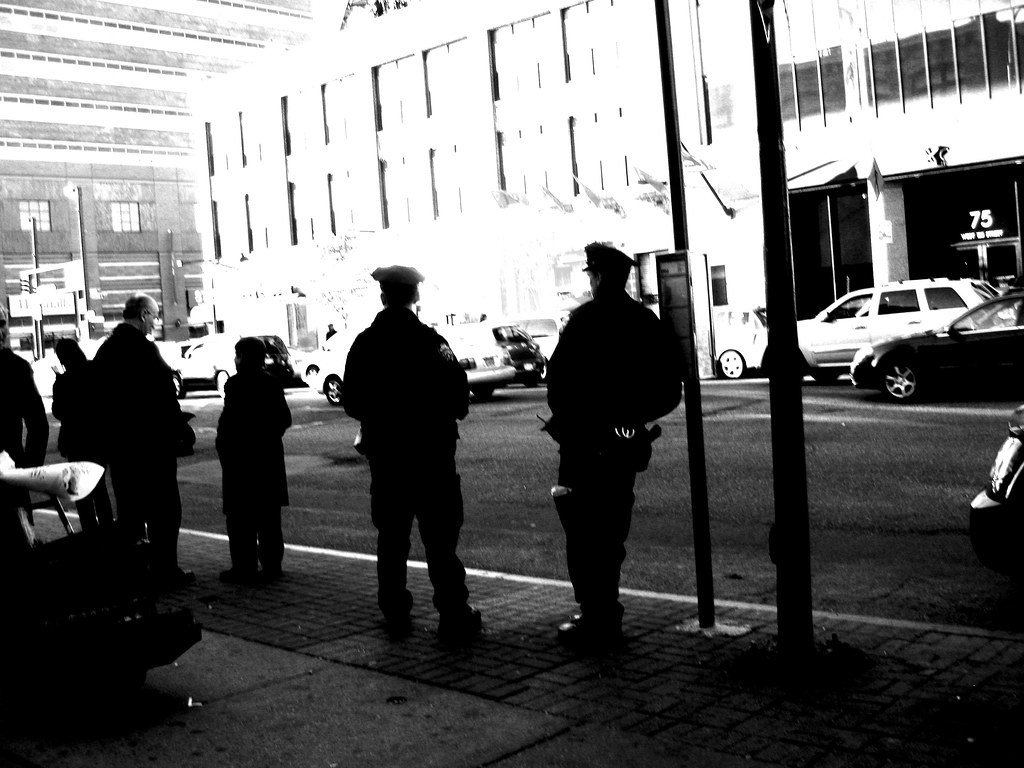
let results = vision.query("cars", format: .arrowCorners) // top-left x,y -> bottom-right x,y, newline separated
967,405 -> 1024,576
299,317 -> 571,399
710,304 -> 769,379
848,294 -> 1024,403
154,334 -> 296,398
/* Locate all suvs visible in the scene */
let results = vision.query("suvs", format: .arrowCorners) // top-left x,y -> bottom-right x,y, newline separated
797,277 -> 1001,383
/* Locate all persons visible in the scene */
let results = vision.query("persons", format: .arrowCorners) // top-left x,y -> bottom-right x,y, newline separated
91,297 -> 197,586
541,243 -> 685,642
215,338 -> 293,585
0,320 -> 50,466
53,339 -> 114,535
339,267 -> 481,639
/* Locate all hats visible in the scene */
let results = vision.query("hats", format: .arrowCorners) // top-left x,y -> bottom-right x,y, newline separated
581,244 -> 637,275
372,265 -> 424,296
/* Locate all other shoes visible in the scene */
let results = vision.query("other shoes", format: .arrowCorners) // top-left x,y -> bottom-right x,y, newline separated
257,546 -> 280,574
152,567 -> 196,582
557,623 -> 615,652
571,613 -> 623,638
437,606 -> 481,634
387,590 -> 413,622
221,567 -> 260,584
